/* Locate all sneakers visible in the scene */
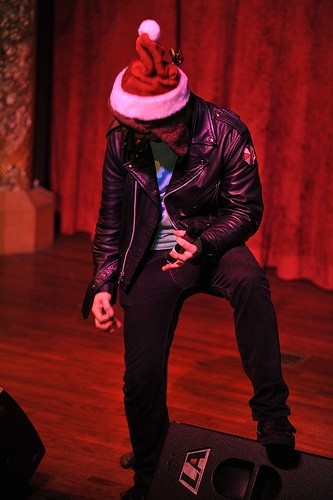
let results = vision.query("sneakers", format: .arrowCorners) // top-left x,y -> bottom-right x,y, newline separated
121,486 -> 145,500
257,415 -> 296,448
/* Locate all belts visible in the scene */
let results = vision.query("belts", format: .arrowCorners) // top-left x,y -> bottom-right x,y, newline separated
151,250 -> 172,258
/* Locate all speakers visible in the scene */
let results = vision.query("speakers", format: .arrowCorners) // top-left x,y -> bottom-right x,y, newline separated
0,387 -> 46,500
146,421 -> 333,500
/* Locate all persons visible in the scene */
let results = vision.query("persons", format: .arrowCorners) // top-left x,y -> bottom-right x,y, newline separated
81,19 -> 303,500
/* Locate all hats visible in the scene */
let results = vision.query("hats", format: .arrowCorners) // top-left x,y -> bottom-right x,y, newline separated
106,19 -> 189,120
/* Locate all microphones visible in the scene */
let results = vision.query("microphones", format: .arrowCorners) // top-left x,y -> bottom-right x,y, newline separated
167,225 -> 204,265
